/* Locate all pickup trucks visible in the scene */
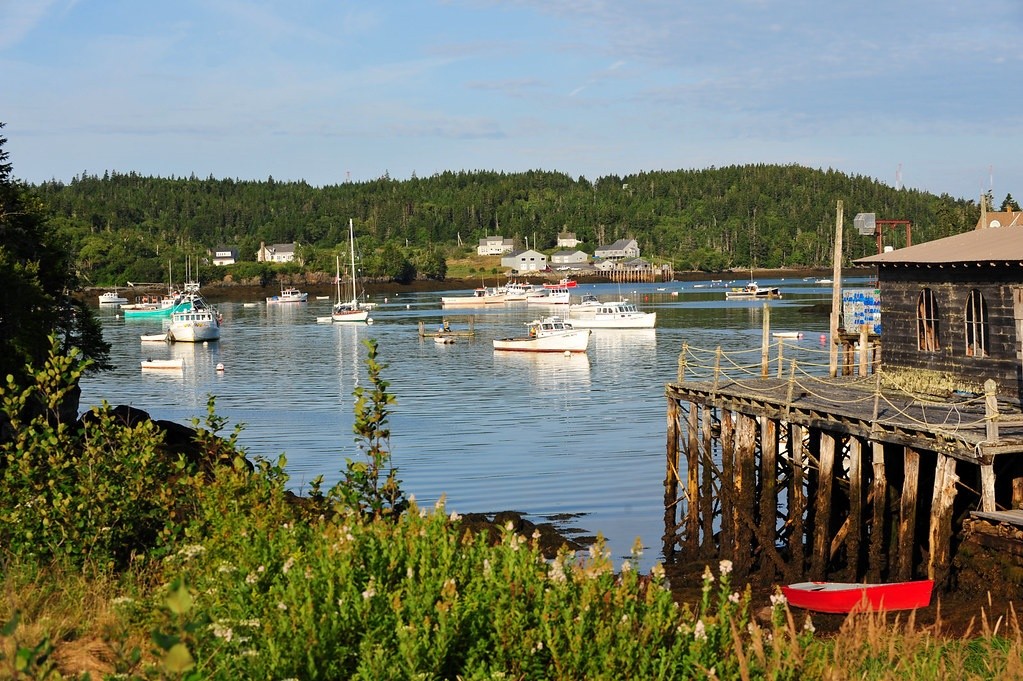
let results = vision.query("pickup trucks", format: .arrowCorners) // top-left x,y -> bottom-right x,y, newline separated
556,265 -> 570,271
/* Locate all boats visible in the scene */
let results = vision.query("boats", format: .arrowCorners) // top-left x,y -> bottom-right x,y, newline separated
317,317 -> 332,320
98,283 -> 129,302
244,302 -> 258,307
266,279 -> 308,303
316,295 -> 330,300
493,314 -> 592,352
694,285 -> 705,287
140,332 -> 167,341
99,302 -> 128,310
657,287 -> 666,291
442,264 -> 577,304
564,278 -> 657,328
125,260 -> 192,321
772,331 -> 799,338
726,269 -> 780,298
170,255 -> 224,341
775,579 -> 934,613
803,275 -> 833,283
140,356 -> 185,369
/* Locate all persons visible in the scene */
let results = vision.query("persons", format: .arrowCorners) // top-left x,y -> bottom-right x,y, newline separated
444,320 -> 451,332
751,287 -> 755,292
745,286 -> 749,292
530,325 -> 538,338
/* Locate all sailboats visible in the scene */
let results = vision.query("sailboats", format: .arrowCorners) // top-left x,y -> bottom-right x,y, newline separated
332,217 -> 368,322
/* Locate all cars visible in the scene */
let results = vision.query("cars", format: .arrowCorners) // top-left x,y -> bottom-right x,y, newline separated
571,267 -> 581,271
